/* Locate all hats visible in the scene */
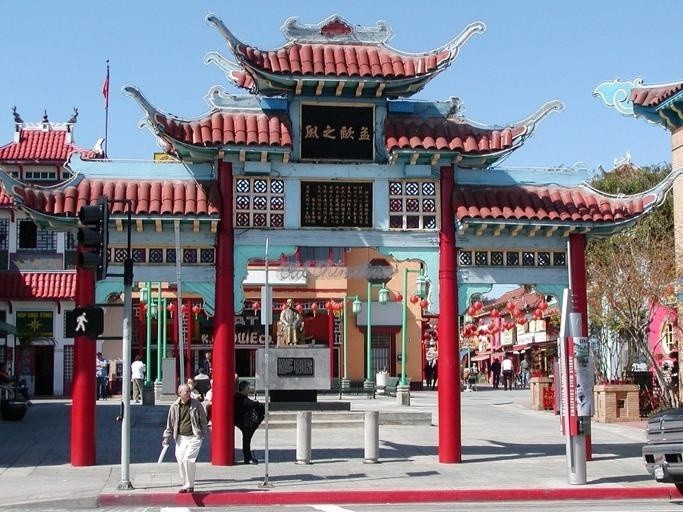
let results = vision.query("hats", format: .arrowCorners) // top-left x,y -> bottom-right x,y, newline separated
494,358 -> 499,361
96,352 -> 102,355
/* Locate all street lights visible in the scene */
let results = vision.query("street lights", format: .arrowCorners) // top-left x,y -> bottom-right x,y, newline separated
343,293 -> 362,379
364,282 -> 389,380
400,267 -> 431,385
138,279 -> 166,387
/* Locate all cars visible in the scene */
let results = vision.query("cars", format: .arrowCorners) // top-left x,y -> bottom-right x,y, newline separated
642,406 -> 682,499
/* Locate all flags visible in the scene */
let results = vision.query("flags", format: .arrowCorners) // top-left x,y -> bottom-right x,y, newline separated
100,58 -> 109,110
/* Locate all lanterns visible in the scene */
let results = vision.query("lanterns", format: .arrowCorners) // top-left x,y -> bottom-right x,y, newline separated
252,303 -> 259,316
311,303 -> 317,318
432,332 -> 437,337
180,304 -> 187,318
395,293 -> 402,302
324,301 -> 331,316
461,298 -> 547,337
423,323 -> 429,330
424,335 -> 430,341
191,305 -> 200,321
331,302 -> 338,316
409,295 -> 418,306
296,305 -> 303,314
433,336 -> 438,342
166,303 -> 175,319
281,304 -> 286,310
335,302 -> 342,314
419,299 -> 428,311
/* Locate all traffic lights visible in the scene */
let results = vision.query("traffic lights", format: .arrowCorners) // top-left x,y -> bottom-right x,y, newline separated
68,308 -> 105,337
75,205 -> 103,271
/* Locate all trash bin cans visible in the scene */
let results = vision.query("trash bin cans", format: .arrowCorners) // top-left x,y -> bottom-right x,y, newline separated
108,381 -> 117,396
625,371 -> 653,418
377,373 -> 389,394
143,388 -> 155,407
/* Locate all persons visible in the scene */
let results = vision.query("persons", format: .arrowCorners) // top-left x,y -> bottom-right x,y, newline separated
129,354 -> 146,403
279,298 -> 303,347
234,379 -> 264,464
201,378 -> 212,427
518,355 -> 530,389
201,351 -> 212,380
500,356 -> 514,390
187,378 -> 202,402
490,357 -> 500,389
95,351 -> 108,400
194,367 -> 209,380
160,384 -> 208,495
432,360 -> 437,390
423,361 -> 432,388
0,362 -> 34,406
469,362 -> 478,374
425,363 -> 432,385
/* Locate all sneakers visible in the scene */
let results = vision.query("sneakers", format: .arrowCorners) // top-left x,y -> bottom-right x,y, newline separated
244,459 -> 258,465
178,488 -> 194,493
